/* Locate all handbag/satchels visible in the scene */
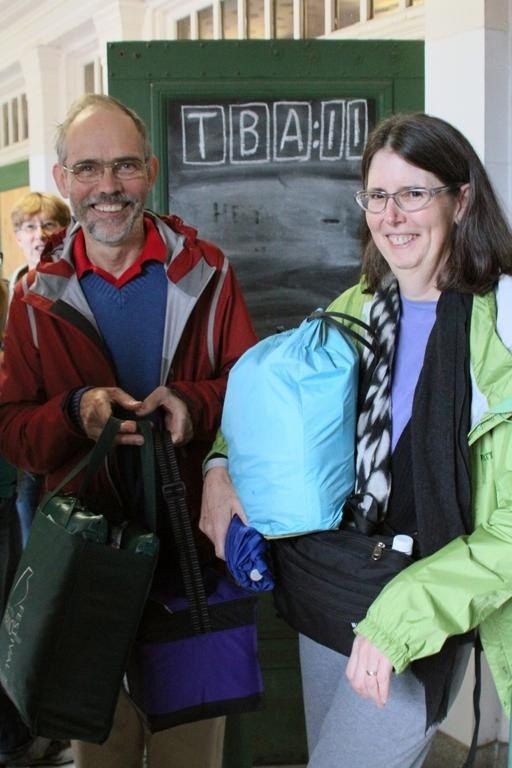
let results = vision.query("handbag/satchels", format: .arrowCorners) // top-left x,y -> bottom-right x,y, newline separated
221,309 -> 380,537
271,490 -> 422,657
126,426 -> 265,732
0,417 -> 161,744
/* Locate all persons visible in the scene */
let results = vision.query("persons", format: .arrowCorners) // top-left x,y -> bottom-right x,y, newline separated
199,117 -> 511,768
0,94 -> 262,766
10,192 -> 72,294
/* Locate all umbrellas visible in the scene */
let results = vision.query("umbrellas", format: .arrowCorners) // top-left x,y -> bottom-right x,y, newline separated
225,512 -> 276,594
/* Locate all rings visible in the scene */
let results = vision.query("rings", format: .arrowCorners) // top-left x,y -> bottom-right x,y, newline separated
365,671 -> 378,680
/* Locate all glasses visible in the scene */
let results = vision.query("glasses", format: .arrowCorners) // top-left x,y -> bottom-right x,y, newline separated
352,186 -> 446,215
66,159 -> 148,184
